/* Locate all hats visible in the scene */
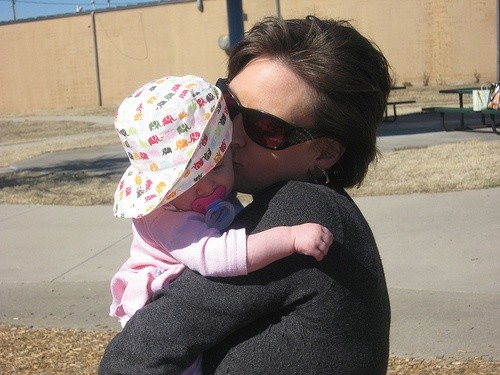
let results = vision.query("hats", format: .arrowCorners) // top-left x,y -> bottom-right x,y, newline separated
112,75 -> 234,218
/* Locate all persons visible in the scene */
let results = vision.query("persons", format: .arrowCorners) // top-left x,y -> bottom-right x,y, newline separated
98,16 -> 391,375
111,76 -> 334,375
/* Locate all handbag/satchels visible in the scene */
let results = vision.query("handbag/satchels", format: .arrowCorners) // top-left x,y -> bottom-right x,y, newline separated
487,82 -> 500,109
473,83 -> 490,111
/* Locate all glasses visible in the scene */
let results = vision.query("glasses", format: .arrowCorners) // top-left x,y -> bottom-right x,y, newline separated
213,77 -> 336,150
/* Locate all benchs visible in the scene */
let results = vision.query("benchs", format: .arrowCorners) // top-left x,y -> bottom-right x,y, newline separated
422,107 -> 500,133
386,100 -> 416,120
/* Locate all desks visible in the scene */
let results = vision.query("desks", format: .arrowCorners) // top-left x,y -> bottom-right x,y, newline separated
439,85 -> 499,129
385,86 -> 406,120
